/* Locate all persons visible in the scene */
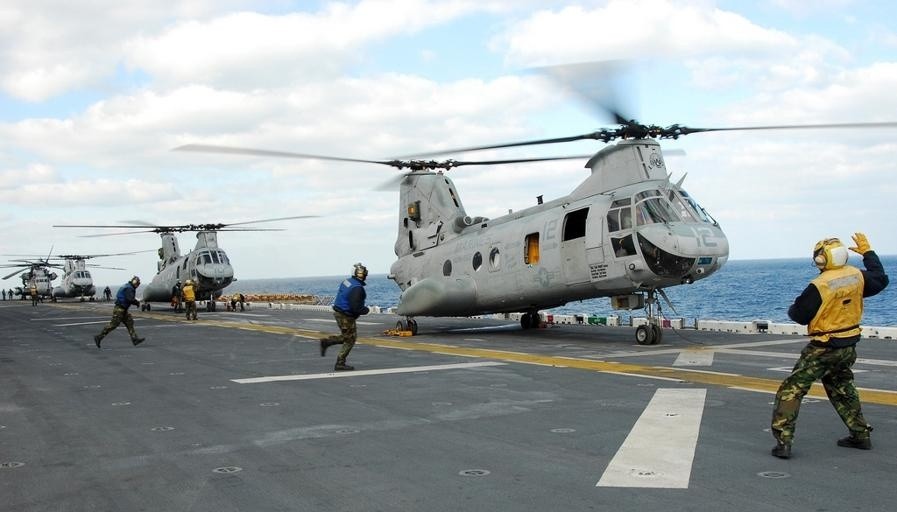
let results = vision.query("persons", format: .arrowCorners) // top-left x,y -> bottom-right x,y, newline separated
92,276 -> 146,349
771,231 -> 889,459
320,261 -> 371,370
623,206 -> 658,258
2,279 -> 245,320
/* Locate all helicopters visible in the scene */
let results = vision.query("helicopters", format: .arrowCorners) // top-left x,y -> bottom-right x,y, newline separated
167,54 -> 895,348
52,208 -> 321,316
0,250 -> 138,304
0,259 -> 99,305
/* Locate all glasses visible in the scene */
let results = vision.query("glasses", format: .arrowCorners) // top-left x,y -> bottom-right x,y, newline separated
137,282 -> 141,286
364,271 -> 369,274
178,281 -> 182,283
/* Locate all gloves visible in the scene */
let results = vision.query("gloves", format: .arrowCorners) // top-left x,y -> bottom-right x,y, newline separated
848,231 -> 870,255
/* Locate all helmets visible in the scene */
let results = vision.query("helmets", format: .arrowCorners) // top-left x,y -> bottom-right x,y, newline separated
354,265 -> 367,279
814,238 -> 847,271
31,286 -> 35,289
185,279 -> 192,285
176,279 -> 181,285
131,277 -> 140,284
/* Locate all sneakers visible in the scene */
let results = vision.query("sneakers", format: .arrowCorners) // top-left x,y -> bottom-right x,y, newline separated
93,335 -> 101,349
835,436 -> 873,448
334,364 -> 355,370
320,338 -> 328,356
771,444 -> 790,457
134,338 -> 144,346
